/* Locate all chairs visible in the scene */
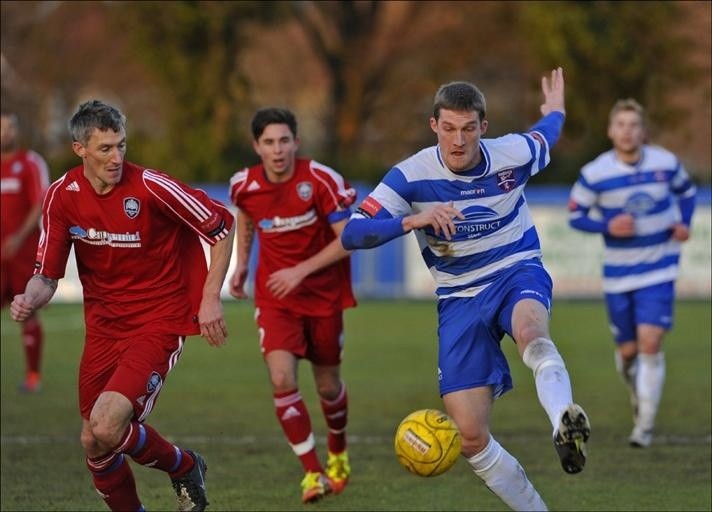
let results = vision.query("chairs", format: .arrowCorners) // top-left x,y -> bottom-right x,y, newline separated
300,467 -> 333,504
626,390 -> 653,449
552,402 -> 592,476
324,450 -> 351,496
170,448 -> 210,512
24,372 -> 43,396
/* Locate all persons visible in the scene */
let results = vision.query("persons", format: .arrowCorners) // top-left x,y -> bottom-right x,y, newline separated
341,66 -> 591,512
9,101 -> 235,512
567,99 -> 696,449
229,106 -> 360,502
0,105 -> 50,394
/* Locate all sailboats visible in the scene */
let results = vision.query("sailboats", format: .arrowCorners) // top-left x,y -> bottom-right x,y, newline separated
394,409 -> 465,479
